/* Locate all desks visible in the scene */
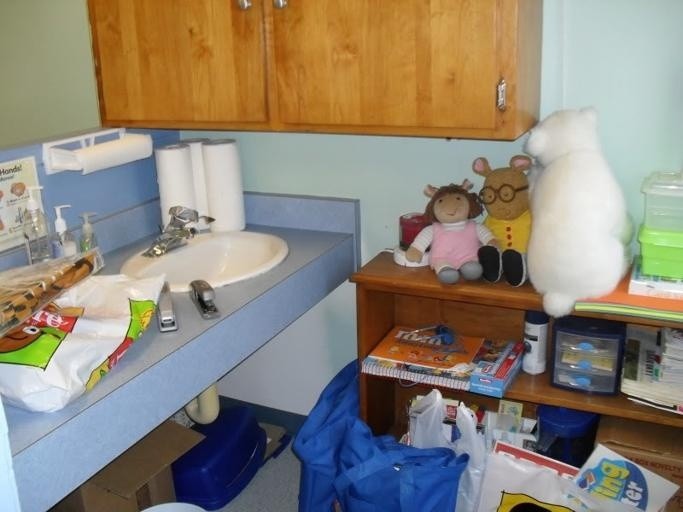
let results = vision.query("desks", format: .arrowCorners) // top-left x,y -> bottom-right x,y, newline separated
0,130 -> 361,512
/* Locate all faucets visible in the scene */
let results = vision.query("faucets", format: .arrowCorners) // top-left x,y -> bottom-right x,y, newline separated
141,205 -> 215,257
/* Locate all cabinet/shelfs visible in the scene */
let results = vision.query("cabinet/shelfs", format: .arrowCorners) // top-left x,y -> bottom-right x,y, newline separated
350,238 -> 681,510
86,0 -> 269,129
268,12 -> 544,143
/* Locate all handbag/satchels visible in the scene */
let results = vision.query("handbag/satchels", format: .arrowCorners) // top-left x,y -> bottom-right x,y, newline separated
292,359 -> 358,512
334,418 -> 470,511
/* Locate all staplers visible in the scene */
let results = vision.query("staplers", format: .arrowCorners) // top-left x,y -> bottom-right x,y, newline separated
157,279 -> 178,332
190,280 -> 220,320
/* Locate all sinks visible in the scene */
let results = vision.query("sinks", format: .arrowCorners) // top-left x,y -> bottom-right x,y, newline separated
119,231 -> 289,293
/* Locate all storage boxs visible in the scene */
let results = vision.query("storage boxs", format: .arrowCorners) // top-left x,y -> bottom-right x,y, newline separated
639,168 -> 681,231
635,228 -> 682,281
591,419 -> 680,512
56,420 -> 205,512
551,320 -> 626,399
